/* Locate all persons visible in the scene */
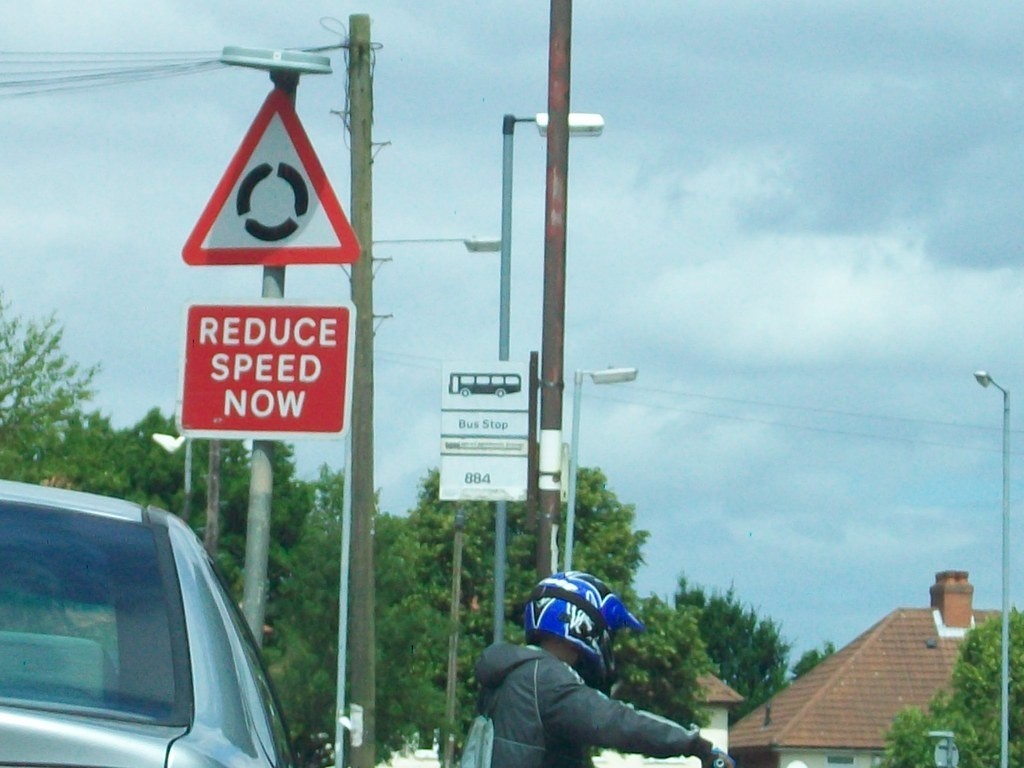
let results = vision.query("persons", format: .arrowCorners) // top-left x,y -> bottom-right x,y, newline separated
462,571 -> 737,768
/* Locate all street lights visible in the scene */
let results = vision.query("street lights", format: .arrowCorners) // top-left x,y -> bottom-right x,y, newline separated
564,364 -> 641,578
465,108 -> 607,650
974,370 -> 1012,768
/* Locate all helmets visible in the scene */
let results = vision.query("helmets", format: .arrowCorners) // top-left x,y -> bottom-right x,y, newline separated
523,570 -> 643,686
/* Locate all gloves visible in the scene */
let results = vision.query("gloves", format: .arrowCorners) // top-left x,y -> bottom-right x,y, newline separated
700,746 -> 736,767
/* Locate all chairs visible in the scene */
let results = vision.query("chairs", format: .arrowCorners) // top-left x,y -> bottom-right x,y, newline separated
0,631 -> 121,706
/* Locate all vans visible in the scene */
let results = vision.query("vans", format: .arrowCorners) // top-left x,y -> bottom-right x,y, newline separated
0,482 -> 297,768
